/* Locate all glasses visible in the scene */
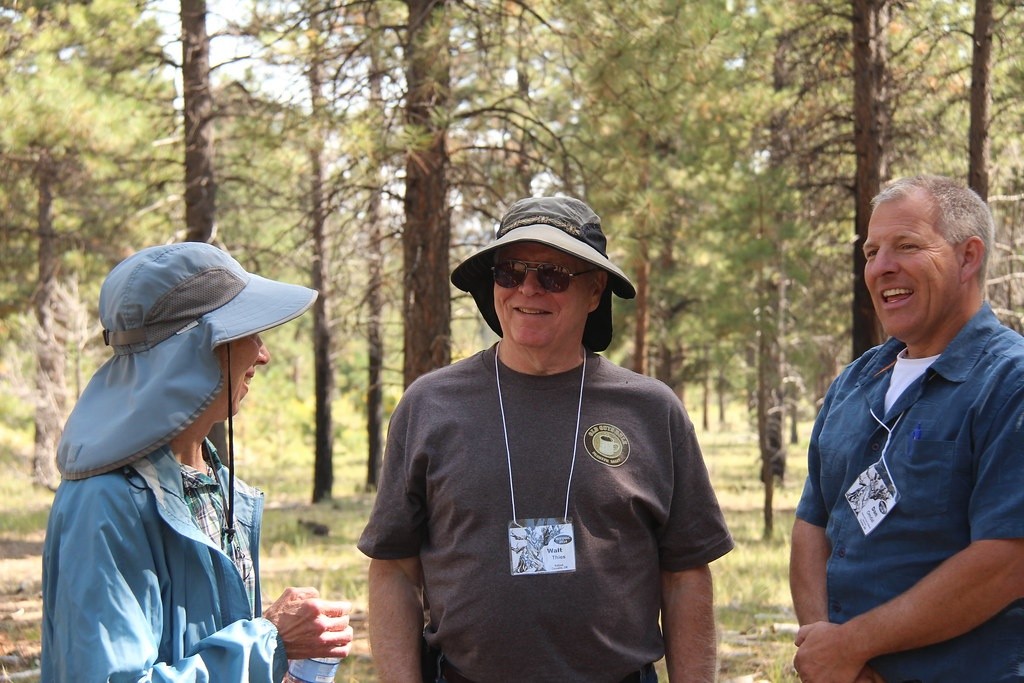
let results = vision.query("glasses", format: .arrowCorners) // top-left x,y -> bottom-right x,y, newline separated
491,259 -> 599,293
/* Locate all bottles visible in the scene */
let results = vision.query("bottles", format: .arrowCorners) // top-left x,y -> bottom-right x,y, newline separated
281,643 -> 342,683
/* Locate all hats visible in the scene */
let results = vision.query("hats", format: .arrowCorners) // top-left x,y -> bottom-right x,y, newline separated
451,197 -> 637,352
55,243 -> 319,480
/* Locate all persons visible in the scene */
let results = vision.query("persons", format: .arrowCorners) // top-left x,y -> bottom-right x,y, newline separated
789,176 -> 1024,683
41,242 -> 353,683
357,195 -> 735,683
